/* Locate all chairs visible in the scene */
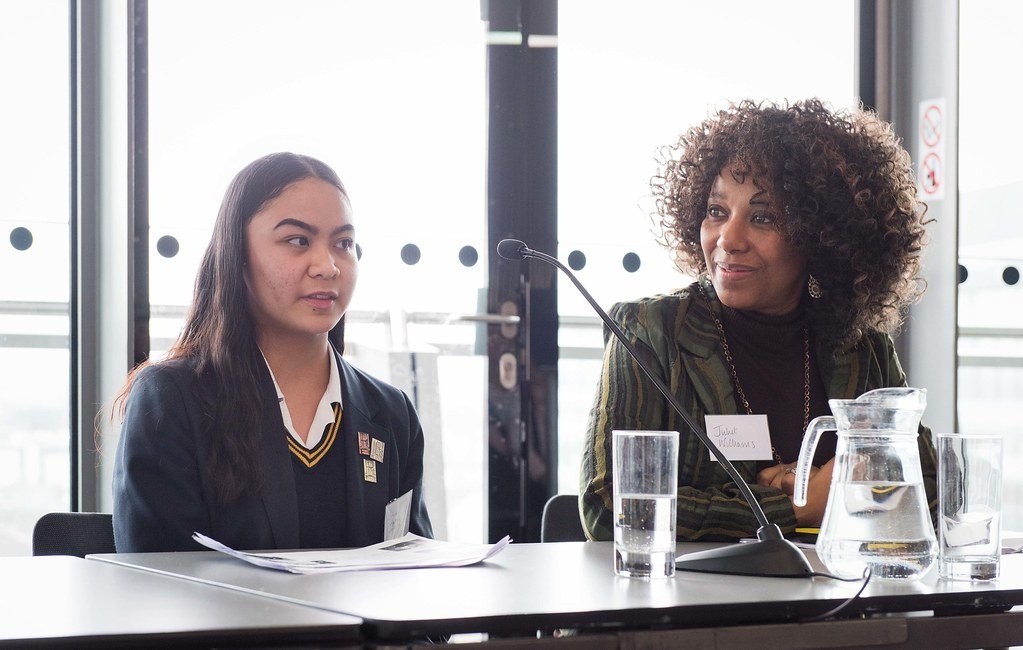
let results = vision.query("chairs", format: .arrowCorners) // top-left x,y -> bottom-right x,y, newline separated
542,494 -> 584,542
33,513 -> 116,558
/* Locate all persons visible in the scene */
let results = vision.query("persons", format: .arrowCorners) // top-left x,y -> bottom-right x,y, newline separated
111,153 -> 451,646
576,96 -> 963,544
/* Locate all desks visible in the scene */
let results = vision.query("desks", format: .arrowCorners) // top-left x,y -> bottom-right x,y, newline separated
86,540 -> 1023,650
1,555 -> 364,650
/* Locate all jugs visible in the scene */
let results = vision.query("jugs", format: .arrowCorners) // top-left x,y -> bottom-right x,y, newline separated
793,387 -> 939,580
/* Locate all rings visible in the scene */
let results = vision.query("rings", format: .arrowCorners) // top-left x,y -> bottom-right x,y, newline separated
786,465 -> 798,476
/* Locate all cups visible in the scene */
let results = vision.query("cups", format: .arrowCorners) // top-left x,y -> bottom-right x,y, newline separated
936,433 -> 1005,584
612,429 -> 679,579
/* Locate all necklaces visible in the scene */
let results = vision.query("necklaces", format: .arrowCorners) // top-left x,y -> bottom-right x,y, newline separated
696,281 -> 810,464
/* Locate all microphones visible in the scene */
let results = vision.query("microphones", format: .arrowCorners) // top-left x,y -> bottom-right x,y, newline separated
497,239 -> 815,577
